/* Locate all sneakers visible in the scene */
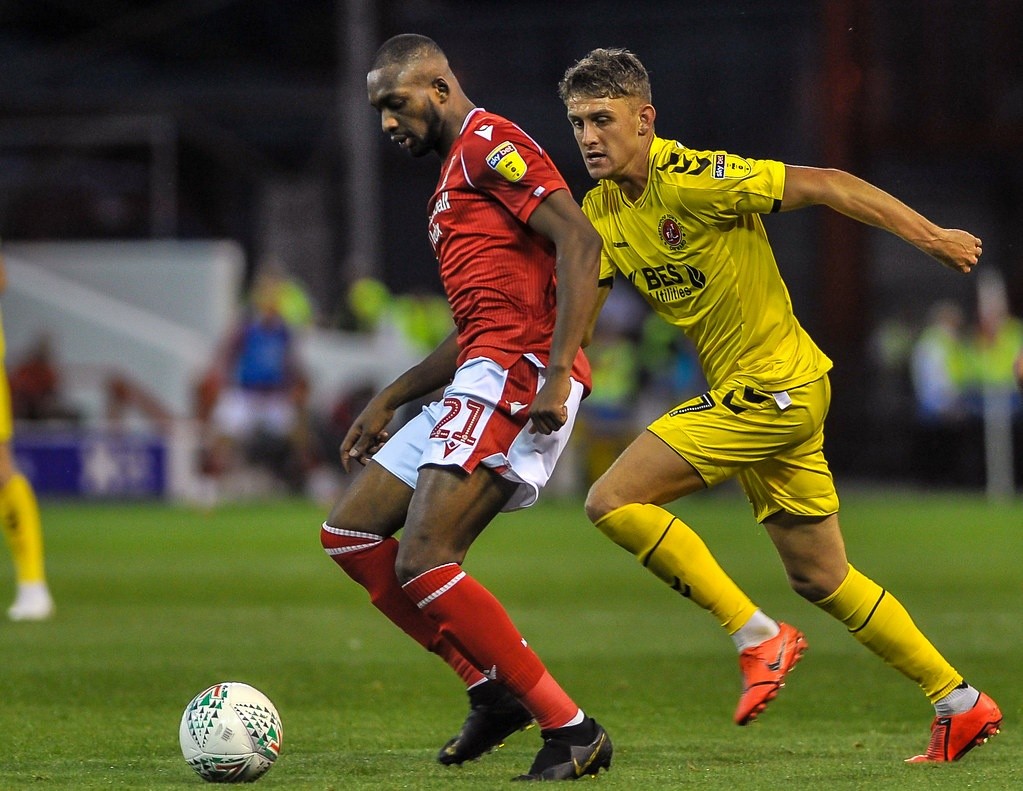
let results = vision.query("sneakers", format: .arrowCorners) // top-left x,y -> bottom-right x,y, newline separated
904,692 -> 1003,763
438,693 -> 535,765
510,718 -> 612,782
735,622 -> 808,726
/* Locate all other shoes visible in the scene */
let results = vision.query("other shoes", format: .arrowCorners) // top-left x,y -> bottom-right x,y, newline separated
9,584 -> 55,619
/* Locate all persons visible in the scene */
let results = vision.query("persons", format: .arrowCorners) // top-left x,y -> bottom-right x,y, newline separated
557,48 -> 1003,764
319,33 -> 613,783
7,251 -> 1023,516
0,251 -> 57,625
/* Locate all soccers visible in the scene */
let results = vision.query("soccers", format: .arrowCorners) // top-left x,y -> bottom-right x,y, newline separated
177,681 -> 283,783
